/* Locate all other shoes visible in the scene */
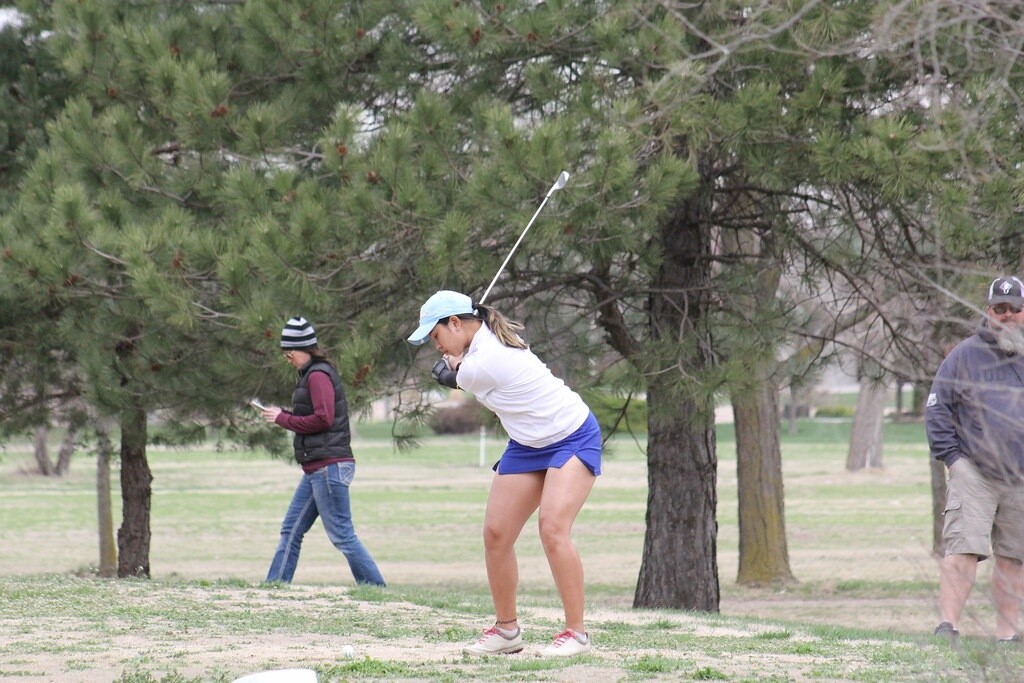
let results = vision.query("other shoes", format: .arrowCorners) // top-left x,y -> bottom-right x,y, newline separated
1002,628 -> 1024,641
935,623 -> 958,638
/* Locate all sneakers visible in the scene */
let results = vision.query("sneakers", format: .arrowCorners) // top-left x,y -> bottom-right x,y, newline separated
461,627 -> 523,658
536,629 -> 590,658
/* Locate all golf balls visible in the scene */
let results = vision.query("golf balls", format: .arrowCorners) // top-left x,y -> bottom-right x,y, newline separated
343,645 -> 353,653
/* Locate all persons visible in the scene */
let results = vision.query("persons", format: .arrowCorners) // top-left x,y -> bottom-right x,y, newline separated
261,316 -> 387,590
406,290 -> 601,659
926,275 -> 1024,648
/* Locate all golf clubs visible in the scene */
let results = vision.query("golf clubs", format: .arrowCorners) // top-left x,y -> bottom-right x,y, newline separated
474,171 -> 570,317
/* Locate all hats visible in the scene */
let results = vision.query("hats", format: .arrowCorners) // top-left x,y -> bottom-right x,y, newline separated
281,316 -> 317,350
987,277 -> 1024,310
408,290 -> 479,346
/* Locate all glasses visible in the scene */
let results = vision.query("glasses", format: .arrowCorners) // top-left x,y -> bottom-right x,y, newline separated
993,304 -> 1020,313
283,351 -> 295,359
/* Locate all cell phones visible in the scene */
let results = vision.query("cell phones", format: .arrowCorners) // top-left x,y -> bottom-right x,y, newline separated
252,402 -> 266,412
